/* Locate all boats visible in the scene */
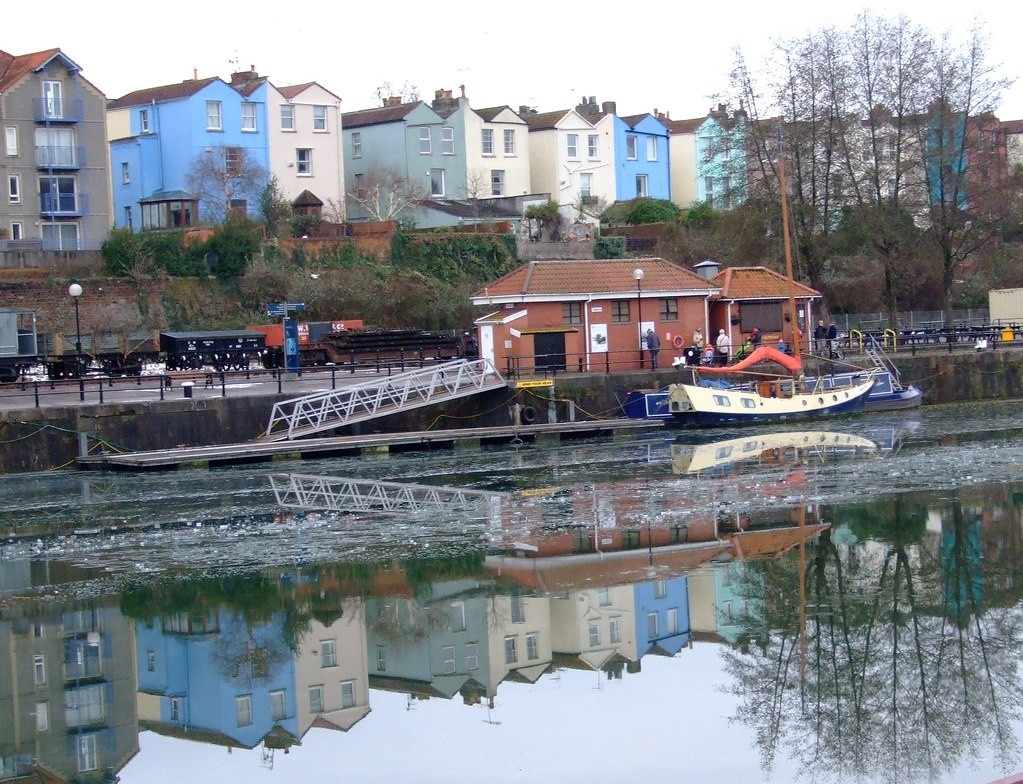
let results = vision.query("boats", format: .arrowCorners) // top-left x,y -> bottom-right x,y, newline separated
624,366 -> 924,424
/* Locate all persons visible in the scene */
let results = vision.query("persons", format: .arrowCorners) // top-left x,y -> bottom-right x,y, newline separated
693,328 -> 703,350
815,320 -> 828,356
778,340 -> 784,353
828,321 -> 837,359
647,329 -> 660,369
700,349 -> 714,365
717,329 -> 730,367
749,327 -> 761,349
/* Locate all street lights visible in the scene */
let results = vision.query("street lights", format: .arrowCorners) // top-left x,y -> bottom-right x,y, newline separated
941,280 -> 963,329
68,283 -> 84,378
634,269 -> 645,368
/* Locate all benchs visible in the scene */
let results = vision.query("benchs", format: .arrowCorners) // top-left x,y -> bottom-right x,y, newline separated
45,361 -> 86,390
164,369 -> 214,392
100,358 -> 142,386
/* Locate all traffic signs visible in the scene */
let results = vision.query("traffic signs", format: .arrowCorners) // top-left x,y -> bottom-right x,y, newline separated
268,303 -> 305,317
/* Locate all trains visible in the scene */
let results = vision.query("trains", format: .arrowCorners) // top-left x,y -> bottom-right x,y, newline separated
0,306 -> 479,383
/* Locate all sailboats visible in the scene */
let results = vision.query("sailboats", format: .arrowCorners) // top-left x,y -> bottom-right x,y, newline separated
670,409 -> 926,719
666,120 -> 875,422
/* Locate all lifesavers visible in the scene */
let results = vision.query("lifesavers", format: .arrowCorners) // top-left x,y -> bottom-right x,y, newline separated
672,335 -> 684,348
522,406 -> 537,422
511,438 -> 523,445
